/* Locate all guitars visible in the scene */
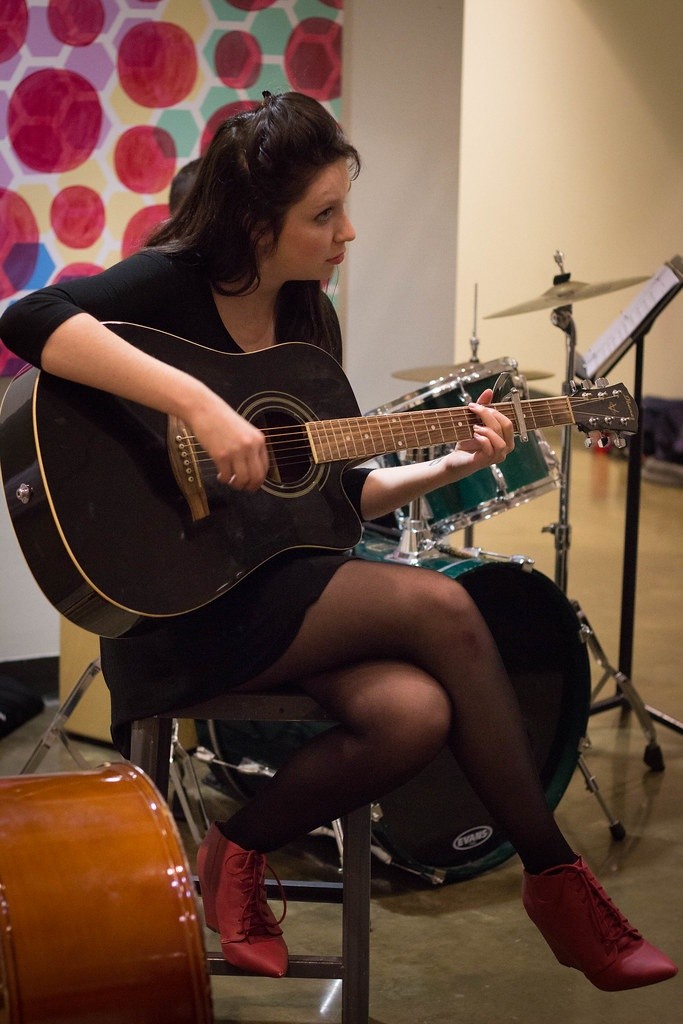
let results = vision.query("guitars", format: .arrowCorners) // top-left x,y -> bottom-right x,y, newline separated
0,321 -> 639,639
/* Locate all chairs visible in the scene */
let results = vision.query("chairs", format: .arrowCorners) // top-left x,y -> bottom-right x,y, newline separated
132,693 -> 373,1024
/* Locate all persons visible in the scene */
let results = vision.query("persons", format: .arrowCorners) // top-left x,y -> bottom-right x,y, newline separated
0,93 -> 677,992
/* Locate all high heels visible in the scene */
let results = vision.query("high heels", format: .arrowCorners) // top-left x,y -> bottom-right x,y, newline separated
196,822 -> 288,979
521,856 -> 679,993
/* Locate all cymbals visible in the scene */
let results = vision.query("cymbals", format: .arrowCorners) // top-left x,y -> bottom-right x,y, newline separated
390,357 -> 556,383
482,272 -> 656,319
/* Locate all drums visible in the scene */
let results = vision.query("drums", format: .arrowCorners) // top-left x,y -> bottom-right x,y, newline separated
193,532 -> 592,890
0,759 -> 215,1024
362,356 -> 561,541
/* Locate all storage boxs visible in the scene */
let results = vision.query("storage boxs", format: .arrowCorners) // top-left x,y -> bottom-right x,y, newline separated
57,615 -> 114,752
